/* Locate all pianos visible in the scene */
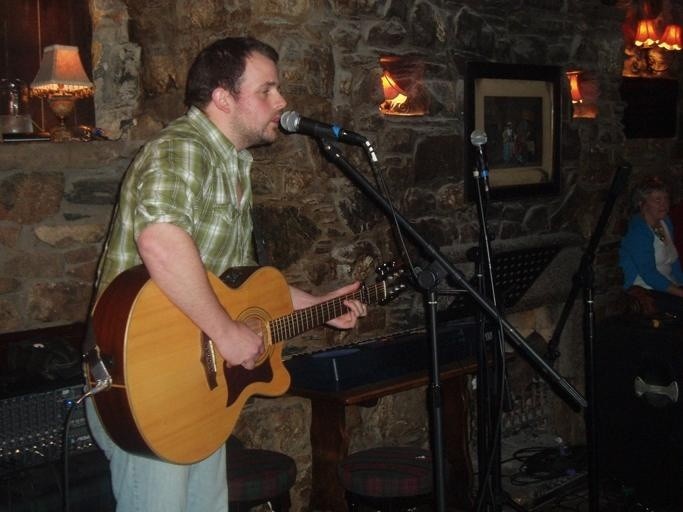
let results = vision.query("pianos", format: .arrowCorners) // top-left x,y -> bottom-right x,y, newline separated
0,322 -> 99,472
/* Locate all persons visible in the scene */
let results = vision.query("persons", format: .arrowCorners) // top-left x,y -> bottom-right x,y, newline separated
83,37 -> 367,512
619,181 -> 683,316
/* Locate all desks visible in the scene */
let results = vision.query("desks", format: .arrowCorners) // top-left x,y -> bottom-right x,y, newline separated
286,352 -> 515,512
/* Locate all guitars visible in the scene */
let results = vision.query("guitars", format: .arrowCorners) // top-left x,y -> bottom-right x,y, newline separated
85,260 -> 408,466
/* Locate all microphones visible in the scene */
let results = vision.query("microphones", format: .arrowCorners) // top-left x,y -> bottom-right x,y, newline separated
470,130 -> 490,192
280,110 -> 368,146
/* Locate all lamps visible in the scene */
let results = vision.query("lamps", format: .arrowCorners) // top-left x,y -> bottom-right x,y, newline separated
612,0 -> 683,78
378,68 -> 411,113
29,44 -> 96,142
566,70 -> 585,105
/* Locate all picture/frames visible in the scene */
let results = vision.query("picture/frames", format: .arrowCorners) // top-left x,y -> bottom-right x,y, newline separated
464,62 -> 563,199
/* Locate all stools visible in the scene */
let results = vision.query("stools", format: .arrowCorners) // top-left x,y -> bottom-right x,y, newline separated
226,450 -> 297,511
338,447 -> 454,512
